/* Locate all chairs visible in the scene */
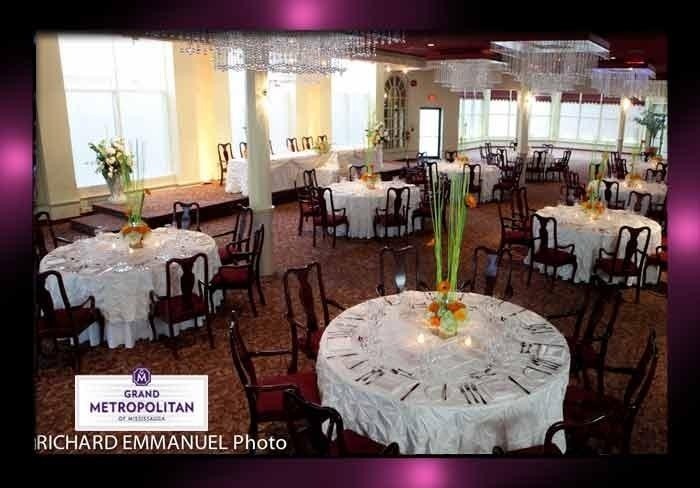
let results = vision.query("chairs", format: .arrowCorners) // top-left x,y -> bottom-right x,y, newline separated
30,134 -> 669,458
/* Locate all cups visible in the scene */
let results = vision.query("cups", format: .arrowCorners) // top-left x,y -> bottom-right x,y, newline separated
362,287 -> 511,388
63,221 -> 194,272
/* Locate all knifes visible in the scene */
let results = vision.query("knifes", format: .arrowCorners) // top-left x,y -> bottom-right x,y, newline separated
399,382 -> 420,402
508,375 -> 530,395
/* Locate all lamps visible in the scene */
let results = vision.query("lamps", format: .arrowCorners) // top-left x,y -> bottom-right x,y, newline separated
129,28 -> 412,82
487,40 -> 610,95
425,59 -> 507,92
589,62 -> 658,107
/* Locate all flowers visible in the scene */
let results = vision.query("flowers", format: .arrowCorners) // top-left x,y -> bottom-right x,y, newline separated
364,120 -> 392,146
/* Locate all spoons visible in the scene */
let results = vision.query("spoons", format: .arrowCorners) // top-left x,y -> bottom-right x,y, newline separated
520,340 -> 526,355
358,368 -> 382,386
458,382 -> 488,406
